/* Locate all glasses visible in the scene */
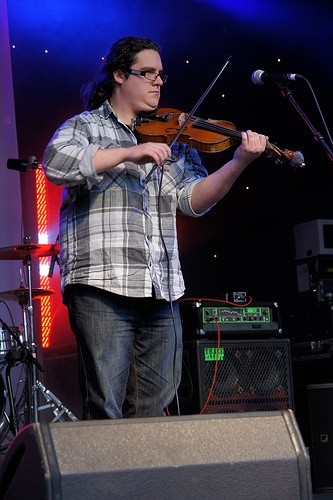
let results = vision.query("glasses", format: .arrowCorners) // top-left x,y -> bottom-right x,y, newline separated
127,68 -> 168,83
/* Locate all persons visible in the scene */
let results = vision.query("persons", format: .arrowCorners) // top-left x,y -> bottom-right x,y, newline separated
41,35 -> 270,421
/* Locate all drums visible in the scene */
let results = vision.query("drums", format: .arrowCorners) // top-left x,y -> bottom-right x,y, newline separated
0,326 -> 24,362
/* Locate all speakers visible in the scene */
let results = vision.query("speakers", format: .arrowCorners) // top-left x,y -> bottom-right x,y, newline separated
0,410 -> 312,500
163,339 -> 294,417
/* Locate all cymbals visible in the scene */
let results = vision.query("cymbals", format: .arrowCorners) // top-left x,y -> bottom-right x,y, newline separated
0,288 -> 54,301
0,243 -> 60,259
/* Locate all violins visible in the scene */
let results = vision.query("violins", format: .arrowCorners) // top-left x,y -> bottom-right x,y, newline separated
135,107 -> 305,170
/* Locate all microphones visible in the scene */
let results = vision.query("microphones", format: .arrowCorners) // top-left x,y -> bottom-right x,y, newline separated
48,237 -> 60,278
251,69 -> 297,87
7,156 -> 39,172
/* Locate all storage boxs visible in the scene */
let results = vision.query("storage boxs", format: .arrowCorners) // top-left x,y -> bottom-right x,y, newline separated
293,219 -> 333,260
